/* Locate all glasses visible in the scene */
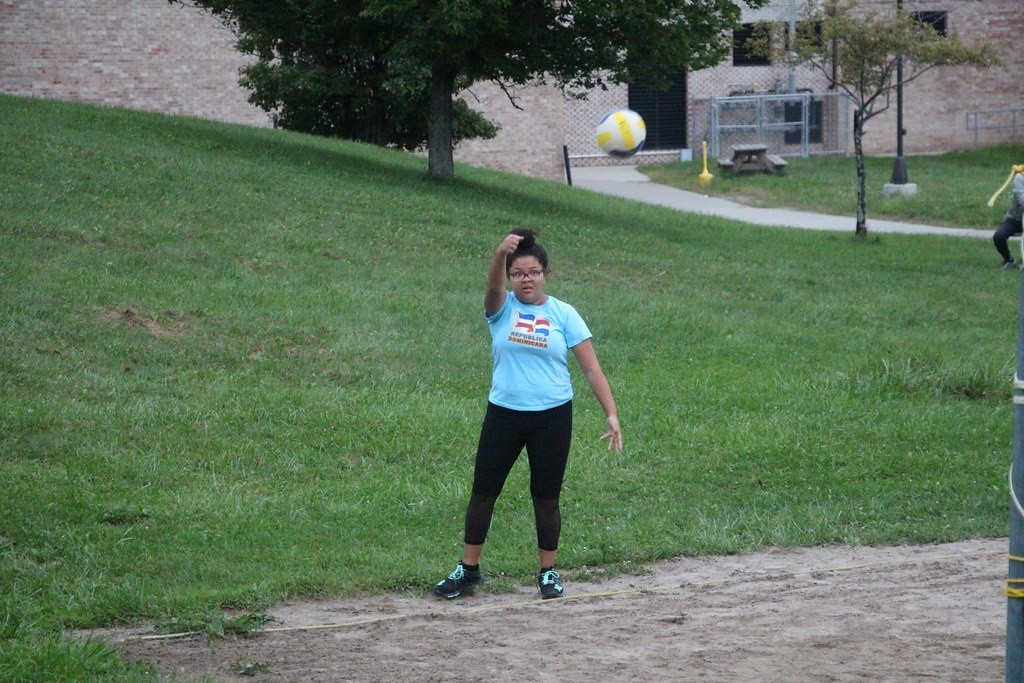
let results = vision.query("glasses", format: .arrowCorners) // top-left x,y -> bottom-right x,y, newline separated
509,269 -> 546,282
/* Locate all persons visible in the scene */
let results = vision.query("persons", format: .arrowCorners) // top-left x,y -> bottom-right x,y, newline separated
993,166 -> 1024,269
433,226 -> 623,600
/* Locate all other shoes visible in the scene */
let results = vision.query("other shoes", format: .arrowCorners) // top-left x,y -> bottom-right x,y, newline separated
1002,258 -> 1015,268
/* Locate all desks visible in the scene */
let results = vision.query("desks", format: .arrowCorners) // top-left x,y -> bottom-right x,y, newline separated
730,144 -> 776,175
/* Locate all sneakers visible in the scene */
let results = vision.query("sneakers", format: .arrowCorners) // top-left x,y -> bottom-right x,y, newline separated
538,568 -> 564,598
432,561 -> 484,600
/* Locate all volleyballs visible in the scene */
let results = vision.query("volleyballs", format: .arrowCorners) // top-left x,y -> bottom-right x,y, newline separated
596,107 -> 648,161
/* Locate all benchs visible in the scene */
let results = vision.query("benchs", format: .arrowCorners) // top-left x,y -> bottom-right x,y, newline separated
718,159 -> 735,167
766,155 -> 788,166
